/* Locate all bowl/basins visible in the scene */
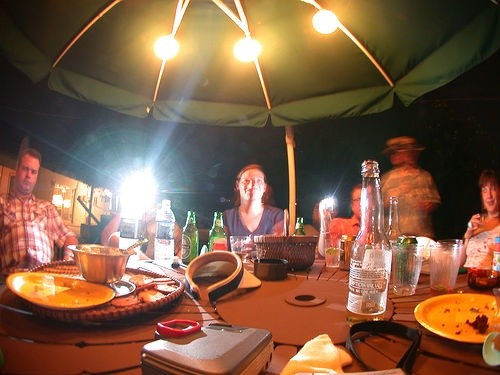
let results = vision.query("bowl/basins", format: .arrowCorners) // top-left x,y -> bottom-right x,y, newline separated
65,242 -> 136,283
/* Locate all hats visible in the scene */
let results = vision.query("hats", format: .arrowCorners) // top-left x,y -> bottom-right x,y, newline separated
383,136 -> 425,154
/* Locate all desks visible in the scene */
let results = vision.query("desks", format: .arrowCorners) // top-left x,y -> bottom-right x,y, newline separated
0,255 -> 500,375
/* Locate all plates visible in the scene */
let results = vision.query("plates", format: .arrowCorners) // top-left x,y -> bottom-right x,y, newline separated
413,293 -> 500,344
6,271 -> 115,309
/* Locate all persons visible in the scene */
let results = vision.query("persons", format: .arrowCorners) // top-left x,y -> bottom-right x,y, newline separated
222,163 -> 287,239
307,137 -> 500,269
0,148 -> 183,283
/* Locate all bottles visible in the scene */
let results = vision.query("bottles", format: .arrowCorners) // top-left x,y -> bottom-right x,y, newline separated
182,211 -> 199,264
118,196 -> 140,268
208,211 -> 228,252
493,237 -> 500,289
292,217 -> 306,236
154,200 -> 175,266
386,196 -> 403,247
346,160 -> 392,327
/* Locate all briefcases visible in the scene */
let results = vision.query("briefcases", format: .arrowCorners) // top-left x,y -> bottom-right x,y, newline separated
140,318 -> 275,375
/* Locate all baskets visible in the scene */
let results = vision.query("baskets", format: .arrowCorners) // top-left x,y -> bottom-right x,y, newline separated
253,209 -> 318,271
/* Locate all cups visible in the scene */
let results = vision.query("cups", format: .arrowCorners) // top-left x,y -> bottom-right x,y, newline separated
230,235 -> 260,265
325,231 -> 355,270
391,238 -> 496,295
